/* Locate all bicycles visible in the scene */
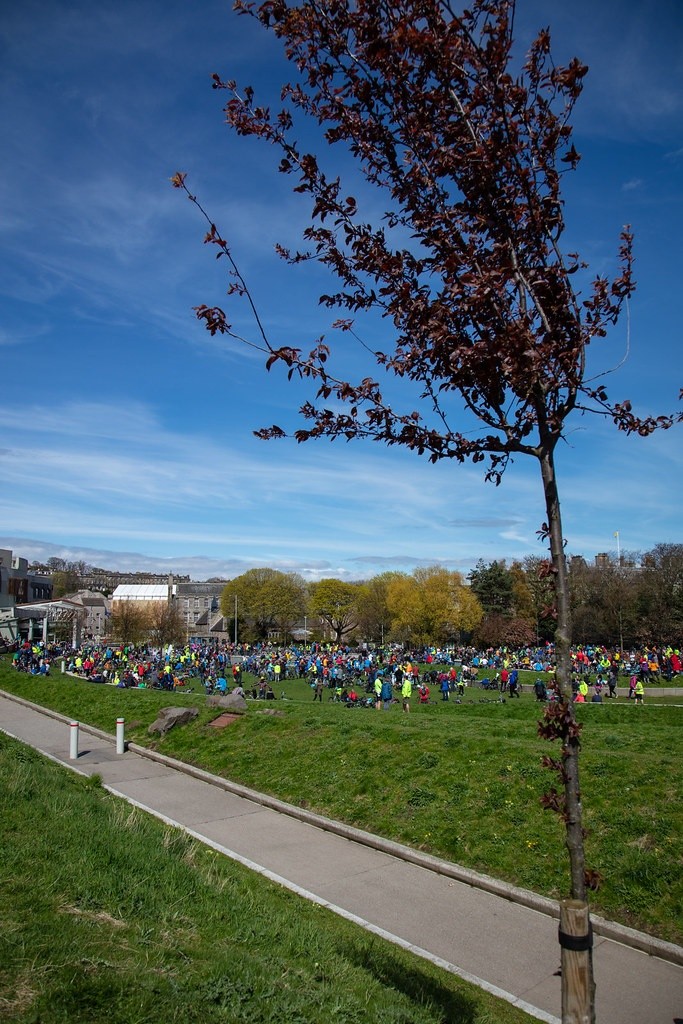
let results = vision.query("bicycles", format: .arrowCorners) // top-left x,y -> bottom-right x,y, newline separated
177,676 -> 190,685
328,692 -> 344,703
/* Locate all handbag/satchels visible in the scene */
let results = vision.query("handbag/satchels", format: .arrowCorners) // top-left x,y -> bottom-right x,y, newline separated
311,683 -> 317,690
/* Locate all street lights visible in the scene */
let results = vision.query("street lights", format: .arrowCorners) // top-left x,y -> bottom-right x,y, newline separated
304,615 -> 308,647
229,595 -> 238,646
185,595 -> 190,642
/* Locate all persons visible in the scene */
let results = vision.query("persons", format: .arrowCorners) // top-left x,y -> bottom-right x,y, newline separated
312,678 -> 322,702
374,674 -> 382,710
508,672 -> 519,698
252,676 -> 271,699
607,672 -> 619,699
0,627 -> 683,704
631,677 -> 644,704
440,677 -> 450,701
449,667 -> 456,692
402,676 -> 411,712
626,674 -> 637,699
83,658 -> 92,677
274,662 -> 281,684
232,663 -> 238,682
204,676 -> 214,695
500,668 -> 510,693
334,665 -> 343,688
594,674 -> 602,695
75,656 -> 82,676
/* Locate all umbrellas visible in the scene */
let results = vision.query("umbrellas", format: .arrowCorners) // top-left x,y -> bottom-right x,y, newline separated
287,628 -> 313,644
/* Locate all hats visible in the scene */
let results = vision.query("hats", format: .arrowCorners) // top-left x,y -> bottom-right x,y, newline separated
209,676 -> 212,680
261,677 -> 265,679
